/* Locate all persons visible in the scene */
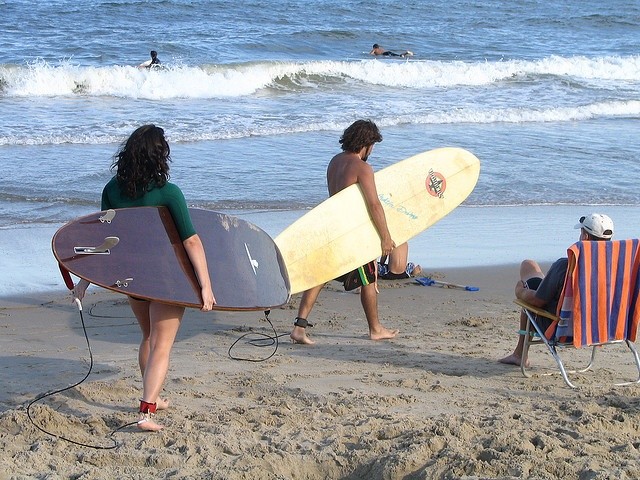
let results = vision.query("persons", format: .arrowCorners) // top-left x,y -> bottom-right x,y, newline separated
379,241 -> 408,280
138,50 -> 160,69
291,120 -> 400,344
369,44 -> 413,58
497,212 -> 614,367
101,125 -> 218,432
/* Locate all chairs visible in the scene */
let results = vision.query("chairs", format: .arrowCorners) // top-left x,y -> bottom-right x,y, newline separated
512,239 -> 640,390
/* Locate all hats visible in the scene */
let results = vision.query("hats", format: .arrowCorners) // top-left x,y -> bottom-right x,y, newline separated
573,213 -> 614,239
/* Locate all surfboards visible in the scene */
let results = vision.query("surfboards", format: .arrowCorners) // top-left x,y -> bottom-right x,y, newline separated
51,206 -> 290,311
273,147 -> 480,296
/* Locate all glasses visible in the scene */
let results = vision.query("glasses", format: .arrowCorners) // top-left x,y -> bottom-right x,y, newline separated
579,216 -> 592,231
